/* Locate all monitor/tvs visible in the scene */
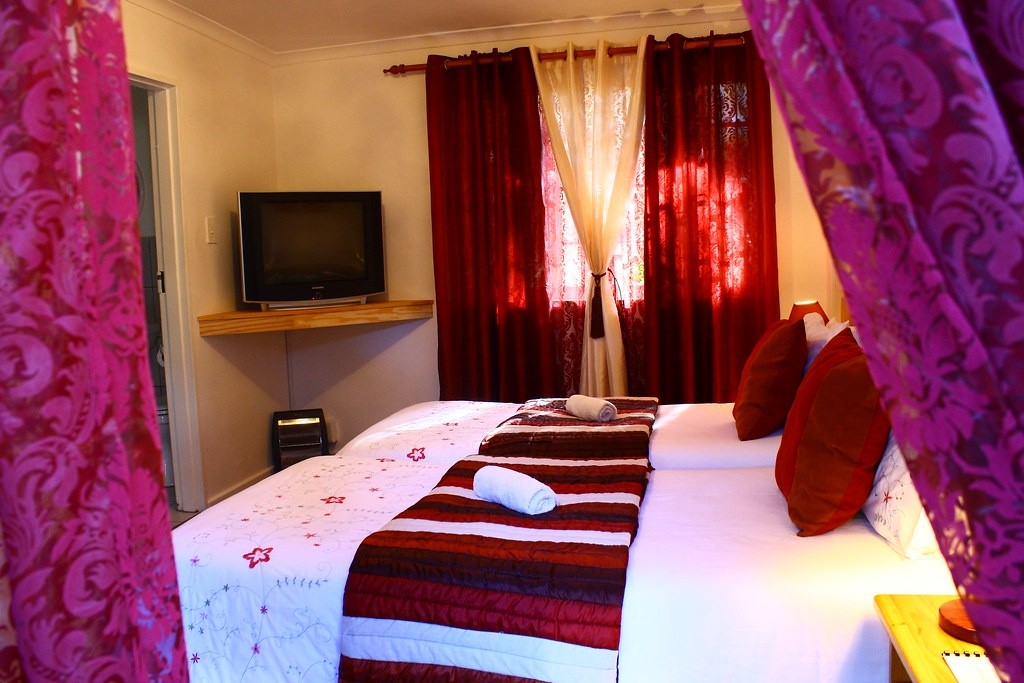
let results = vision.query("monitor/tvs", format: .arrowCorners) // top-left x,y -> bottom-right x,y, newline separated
237,190 -> 387,303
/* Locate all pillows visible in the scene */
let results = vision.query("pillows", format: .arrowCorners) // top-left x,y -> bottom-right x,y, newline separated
770,317 -> 887,540
730,316 -> 809,443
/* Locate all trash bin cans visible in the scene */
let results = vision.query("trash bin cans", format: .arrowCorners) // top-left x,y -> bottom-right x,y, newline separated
271,407 -> 328,471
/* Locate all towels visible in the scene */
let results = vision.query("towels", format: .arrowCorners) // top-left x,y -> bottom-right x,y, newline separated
565,394 -> 618,424
472,465 -> 560,515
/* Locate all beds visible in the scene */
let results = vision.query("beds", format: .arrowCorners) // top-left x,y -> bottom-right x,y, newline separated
161,307 -> 956,679
